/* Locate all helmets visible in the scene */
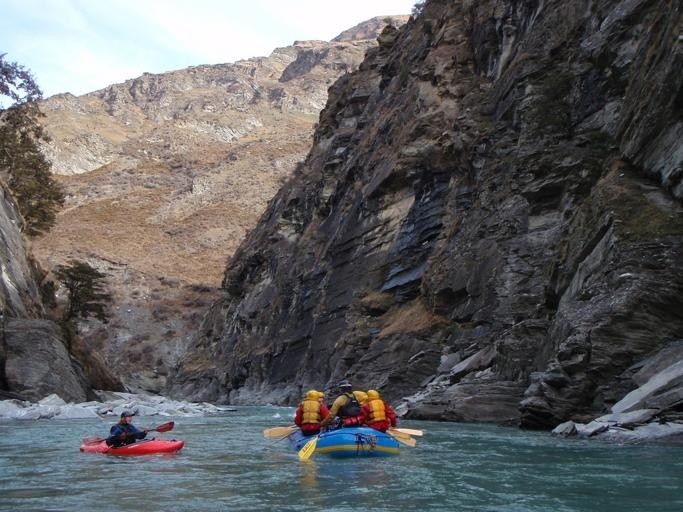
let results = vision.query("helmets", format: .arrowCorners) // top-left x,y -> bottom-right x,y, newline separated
304,389 -> 325,401
352,390 -> 379,404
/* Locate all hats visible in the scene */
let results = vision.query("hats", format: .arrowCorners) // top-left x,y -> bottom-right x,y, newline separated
337,380 -> 353,388
121,412 -> 136,417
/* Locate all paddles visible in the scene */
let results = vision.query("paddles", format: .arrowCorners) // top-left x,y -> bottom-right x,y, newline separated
264,427 -> 300,437
298,430 -> 322,460
385,431 -> 416,447
392,429 -> 423,436
83,422 -> 174,446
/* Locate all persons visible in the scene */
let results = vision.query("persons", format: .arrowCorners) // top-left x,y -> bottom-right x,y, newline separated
293,379 -> 399,433
106,411 -> 150,448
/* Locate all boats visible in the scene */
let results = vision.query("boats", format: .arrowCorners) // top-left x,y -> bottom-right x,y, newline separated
289,428 -> 400,457
80,437 -> 183,456
